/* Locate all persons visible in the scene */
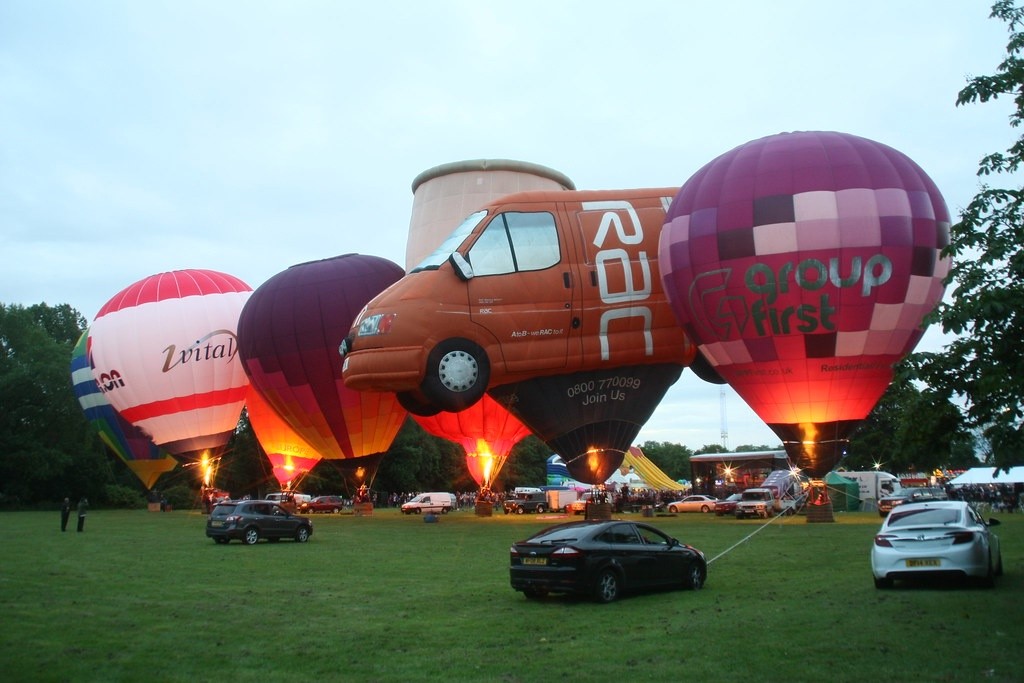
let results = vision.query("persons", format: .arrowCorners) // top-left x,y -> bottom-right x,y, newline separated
209,467 -> 1024,541
76,496 -> 91,533
159,493 -> 168,514
59,497 -> 73,531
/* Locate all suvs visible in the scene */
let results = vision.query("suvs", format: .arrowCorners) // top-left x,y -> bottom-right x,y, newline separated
300,496 -> 345,514
205,500 -> 313,545
400,491 -> 458,515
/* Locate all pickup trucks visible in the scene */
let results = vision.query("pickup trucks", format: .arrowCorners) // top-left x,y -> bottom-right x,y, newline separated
733,487 -> 797,521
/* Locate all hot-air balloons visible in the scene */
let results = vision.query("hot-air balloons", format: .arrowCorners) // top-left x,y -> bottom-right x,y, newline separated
333,185 -> 738,521
67,325 -> 178,512
399,156 -> 583,520
244,386 -> 323,515
85,268 -> 255,517
653,129 -> 955,526
236,252 -> 411,514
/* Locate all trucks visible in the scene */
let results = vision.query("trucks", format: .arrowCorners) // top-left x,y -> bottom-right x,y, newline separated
834,470 -> 903,502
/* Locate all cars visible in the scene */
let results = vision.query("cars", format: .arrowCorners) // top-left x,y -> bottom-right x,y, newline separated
503,491 -> 550,515
715,494 -> 746,516
572,491 -> 614,515
666,494 -> 721,514
869,501 -> 1004,589
508,521 -> 709,603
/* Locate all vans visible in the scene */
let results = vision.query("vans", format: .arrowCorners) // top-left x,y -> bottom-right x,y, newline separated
514,486 -> 542,496
265,492 -> 312,508
878,486 -> 951,518
